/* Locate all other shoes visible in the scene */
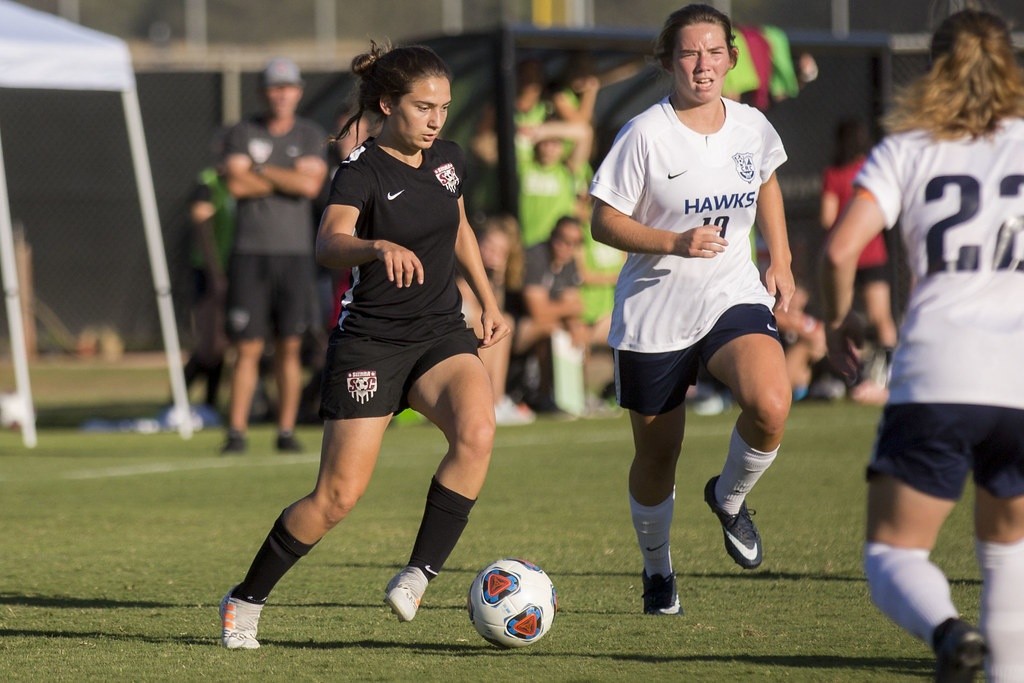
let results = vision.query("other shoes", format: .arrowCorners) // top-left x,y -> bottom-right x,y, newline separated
222,432 -> 246,454
277,434 -> 303,452
495,396 -> 537,426
931,619 -> 988,683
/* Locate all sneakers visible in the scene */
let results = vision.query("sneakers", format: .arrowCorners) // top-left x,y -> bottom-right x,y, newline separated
640,568 -> 681,615
384,565 -> 428,622
704,476 -> 761,568
217,589 -> 264,650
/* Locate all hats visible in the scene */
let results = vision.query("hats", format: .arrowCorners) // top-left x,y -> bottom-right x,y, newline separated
262,58 -> 303,86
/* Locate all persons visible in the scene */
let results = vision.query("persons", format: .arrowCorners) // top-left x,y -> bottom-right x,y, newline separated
185,59 -> 379,454
821,10 -> 1024,683
222,39 -> 513,651
457,74 -> 633,423
590,7 -> 796,615
689,122 -> 903,416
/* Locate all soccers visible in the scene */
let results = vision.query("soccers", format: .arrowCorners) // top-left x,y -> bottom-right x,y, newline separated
467,557 -> 559,650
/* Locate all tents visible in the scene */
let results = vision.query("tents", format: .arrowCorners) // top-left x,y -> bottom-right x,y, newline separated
0,0 -> 192,446
